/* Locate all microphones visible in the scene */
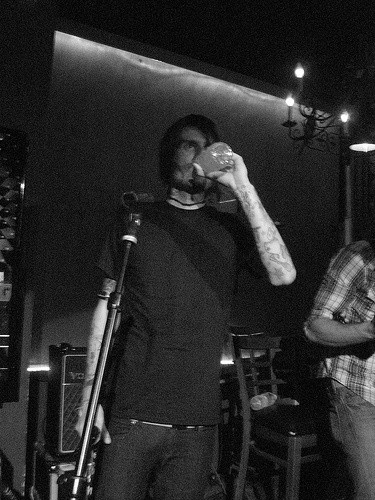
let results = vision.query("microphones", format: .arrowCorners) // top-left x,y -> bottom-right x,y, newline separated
121,191 -> 156,209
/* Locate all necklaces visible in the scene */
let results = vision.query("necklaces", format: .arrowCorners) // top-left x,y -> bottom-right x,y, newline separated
167,195 -> 206,206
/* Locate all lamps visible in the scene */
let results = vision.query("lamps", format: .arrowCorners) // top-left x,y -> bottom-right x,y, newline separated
282,62 -> 375,159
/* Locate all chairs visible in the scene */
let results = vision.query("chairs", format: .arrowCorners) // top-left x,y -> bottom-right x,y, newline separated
227,324 -> 323,500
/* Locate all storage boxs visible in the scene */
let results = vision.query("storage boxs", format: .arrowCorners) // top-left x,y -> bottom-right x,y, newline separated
29,443 -> 87,500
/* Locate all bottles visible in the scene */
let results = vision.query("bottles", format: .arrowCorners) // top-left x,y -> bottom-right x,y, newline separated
193,141 -> 234,174
34,441 -> 57,465
249,393 -> 278,411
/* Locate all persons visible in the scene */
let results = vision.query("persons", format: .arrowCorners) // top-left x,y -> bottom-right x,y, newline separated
302,240 -> 375,500
74,115 -> 297,500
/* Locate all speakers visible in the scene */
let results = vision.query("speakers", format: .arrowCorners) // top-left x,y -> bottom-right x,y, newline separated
45,345 -> 89,457
